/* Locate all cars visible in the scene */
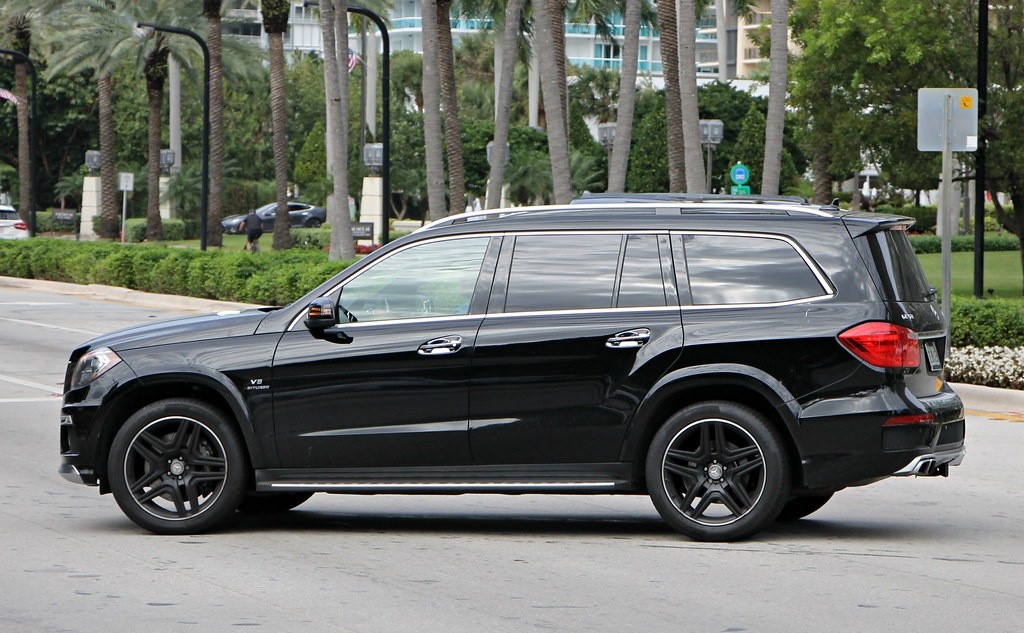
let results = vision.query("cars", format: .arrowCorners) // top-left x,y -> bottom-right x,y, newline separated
856,171 -> 880,200
0,204 -> 29,240
220,200 -> 326,235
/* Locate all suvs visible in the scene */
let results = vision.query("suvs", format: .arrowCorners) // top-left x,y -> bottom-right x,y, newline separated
56,191 -> 967,542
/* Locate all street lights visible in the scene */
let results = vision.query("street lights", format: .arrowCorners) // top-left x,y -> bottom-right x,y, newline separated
137,22 -> 210,252
303,0 -> 390,248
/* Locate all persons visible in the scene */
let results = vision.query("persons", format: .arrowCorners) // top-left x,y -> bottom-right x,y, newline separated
240,209 -> 263,252
